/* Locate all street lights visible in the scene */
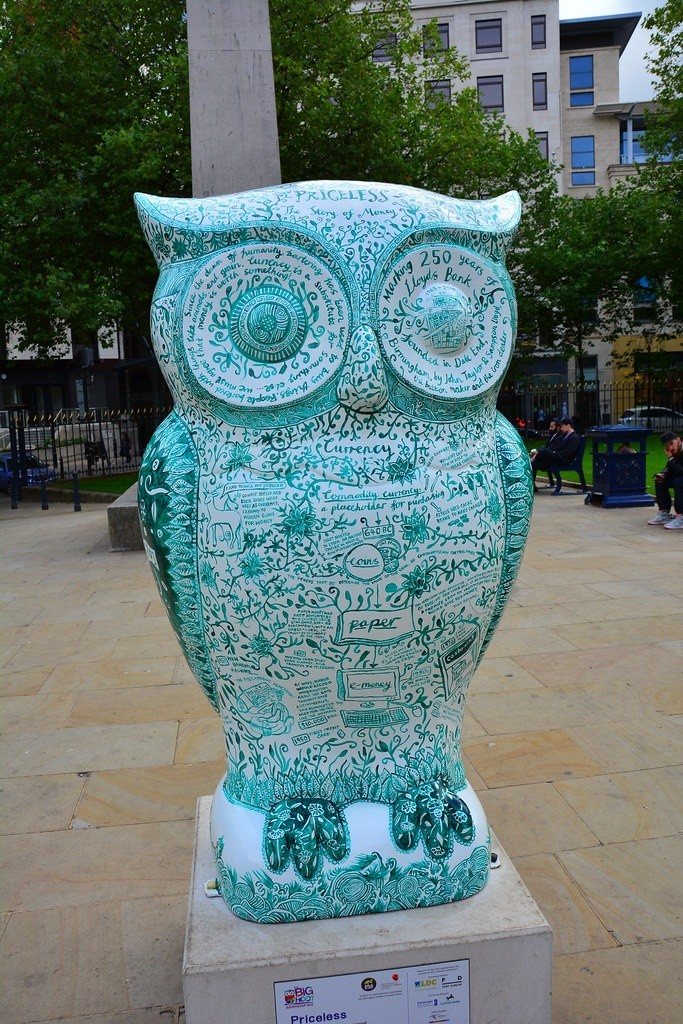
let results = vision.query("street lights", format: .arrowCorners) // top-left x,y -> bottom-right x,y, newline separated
641,327 -> 658,431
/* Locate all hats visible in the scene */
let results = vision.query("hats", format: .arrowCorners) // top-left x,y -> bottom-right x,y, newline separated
660,430 -> 679,446
555,416 -> 572,425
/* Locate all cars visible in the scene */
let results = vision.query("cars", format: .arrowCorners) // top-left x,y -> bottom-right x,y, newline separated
0,452 -> 60,496
618,405 -> 683,432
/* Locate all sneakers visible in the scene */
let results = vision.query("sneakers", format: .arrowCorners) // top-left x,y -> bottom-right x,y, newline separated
647,510 -> 674,525
664,514 -> 683,529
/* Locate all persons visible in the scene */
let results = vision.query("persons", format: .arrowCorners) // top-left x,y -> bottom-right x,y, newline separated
120,431 -> 132,463
647,430 -> 683,530
528,417 -> 581,493
